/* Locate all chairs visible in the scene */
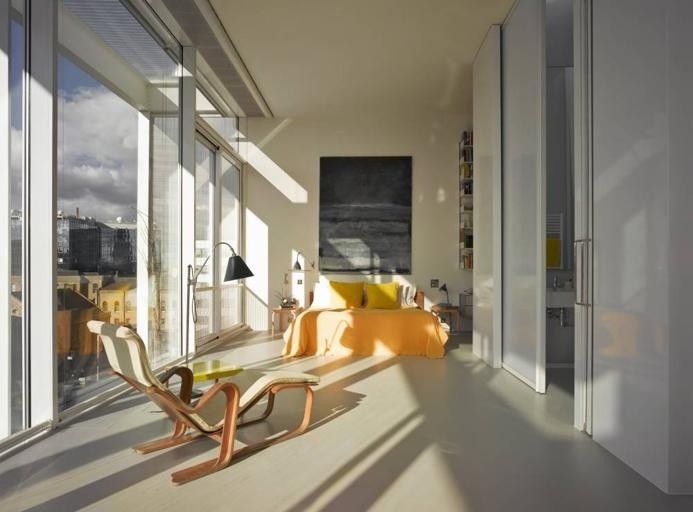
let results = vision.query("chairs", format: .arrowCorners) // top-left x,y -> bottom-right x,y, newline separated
85,320 -> 320,485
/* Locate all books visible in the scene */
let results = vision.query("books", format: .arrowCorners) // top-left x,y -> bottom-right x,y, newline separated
460,130 -> 473,269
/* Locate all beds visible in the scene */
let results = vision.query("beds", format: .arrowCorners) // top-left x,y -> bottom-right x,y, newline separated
279,290 -> 449,360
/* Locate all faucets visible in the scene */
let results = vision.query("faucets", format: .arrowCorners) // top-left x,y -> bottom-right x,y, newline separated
553,276 -> 558,288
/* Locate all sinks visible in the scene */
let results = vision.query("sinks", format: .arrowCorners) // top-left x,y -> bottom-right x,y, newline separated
546,287 -> 576,308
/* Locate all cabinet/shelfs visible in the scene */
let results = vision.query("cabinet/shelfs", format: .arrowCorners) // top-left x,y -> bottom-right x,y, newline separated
457,127 -> 473,272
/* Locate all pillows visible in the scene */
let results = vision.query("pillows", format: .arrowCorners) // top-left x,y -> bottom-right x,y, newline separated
311,280 -> 419,309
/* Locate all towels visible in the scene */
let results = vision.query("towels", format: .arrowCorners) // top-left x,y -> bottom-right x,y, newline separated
545,237 -> 561,270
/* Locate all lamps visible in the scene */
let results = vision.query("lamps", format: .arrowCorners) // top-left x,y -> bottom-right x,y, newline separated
288,252 -> 314,272
280,273 -> 293,308
178,242 -> 254,364
439,284 -> 452,306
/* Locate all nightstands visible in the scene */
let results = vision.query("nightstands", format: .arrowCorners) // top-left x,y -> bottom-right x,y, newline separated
429,306 -> 460,336
270,307 -> 303,340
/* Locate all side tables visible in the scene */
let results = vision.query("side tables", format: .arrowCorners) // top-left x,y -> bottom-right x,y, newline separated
164,359 -> 244,399
